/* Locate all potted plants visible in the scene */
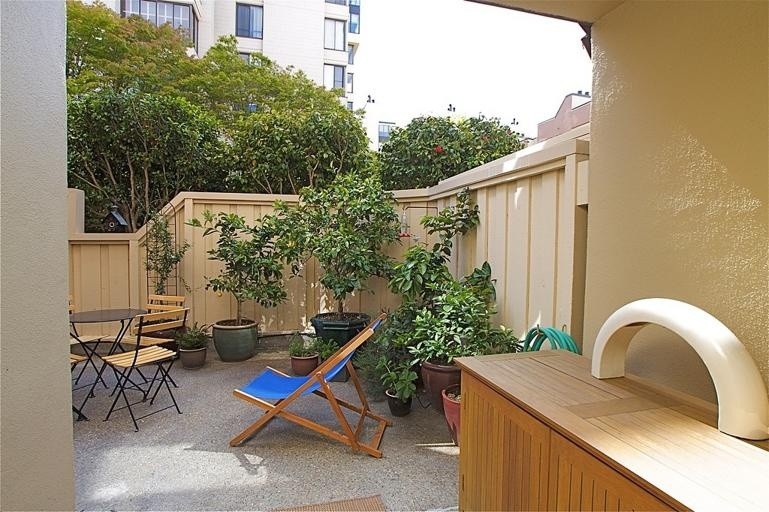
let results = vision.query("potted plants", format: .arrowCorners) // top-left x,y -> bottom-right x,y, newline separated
136,206 -> 185,363
186,203 -> 286,363
279,170 -> 404,353
287,330 -> 322,375
420,278 -> 495,413
177,320 -> 212,369
318,337 -> 351,383
376,348 -> 417,415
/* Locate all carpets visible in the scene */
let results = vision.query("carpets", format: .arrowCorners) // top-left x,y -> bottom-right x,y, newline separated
272,493 -> 386,512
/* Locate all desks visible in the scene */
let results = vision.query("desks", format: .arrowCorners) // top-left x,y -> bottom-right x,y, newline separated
69,310 -> 149,399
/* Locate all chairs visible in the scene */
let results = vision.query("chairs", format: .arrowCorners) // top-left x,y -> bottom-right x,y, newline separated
100,308 -> 191,433
103,293 -> 186,387
69,295 -> 109,389
70,353 -> 90,421
230,314 -> 393,458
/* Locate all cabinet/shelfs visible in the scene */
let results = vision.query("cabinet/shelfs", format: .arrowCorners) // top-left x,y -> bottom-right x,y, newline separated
453,349 -> 769,512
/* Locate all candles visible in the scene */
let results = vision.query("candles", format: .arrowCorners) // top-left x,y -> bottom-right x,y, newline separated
441,324 -> 527,445
